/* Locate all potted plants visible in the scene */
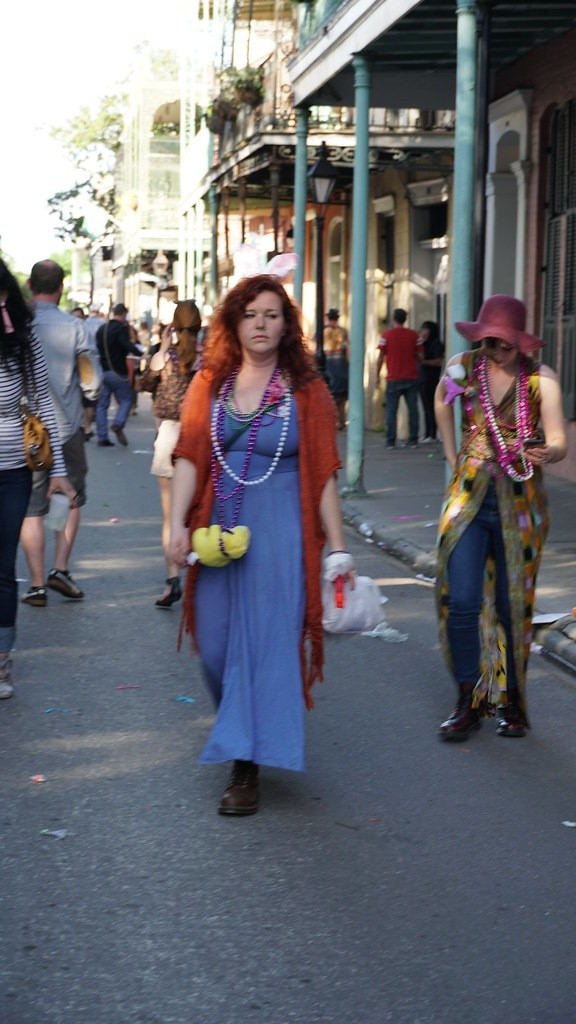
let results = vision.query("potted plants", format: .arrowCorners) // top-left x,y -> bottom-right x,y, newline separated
204,62 -> 265,135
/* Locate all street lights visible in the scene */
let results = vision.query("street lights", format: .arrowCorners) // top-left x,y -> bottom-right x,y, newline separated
153,246 -> 171,319
309,141 -> 340,389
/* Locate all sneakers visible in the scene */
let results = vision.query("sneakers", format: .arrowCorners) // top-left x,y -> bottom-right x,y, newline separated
17,585 -> 48,605
49,568 -> 84,599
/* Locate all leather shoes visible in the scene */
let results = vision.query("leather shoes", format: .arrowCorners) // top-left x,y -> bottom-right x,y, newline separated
217,760 -> 260,815
496,689 -> 525,736
438,681 -> 486,741
152,576 -> 181,607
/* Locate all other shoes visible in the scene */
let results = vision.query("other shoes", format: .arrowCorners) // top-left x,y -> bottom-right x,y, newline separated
423,437 -> 436,444
110,424 -> 126,445
0,653 -> 15,697
99,440 -> 114,448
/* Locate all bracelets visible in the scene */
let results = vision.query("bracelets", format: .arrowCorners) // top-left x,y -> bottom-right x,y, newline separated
327,550 -> 349,557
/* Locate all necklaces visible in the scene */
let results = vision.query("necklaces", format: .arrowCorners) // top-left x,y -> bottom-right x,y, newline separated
211,359 -> 292,528
473,352 -> 533,481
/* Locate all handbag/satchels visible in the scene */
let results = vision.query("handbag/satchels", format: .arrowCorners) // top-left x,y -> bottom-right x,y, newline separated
24,418 -> 52,472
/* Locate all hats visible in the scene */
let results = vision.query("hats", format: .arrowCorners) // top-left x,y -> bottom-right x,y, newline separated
453,294 -> 547,352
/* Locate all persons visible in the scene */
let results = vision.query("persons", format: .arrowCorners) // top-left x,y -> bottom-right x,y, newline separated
375,309 -> 428,451
149,298 -> 208,607
169,271 -> 356,816
434,294 -> 566,739
70,307 -> 96,439
418,320 -> 445,445
0,258 -> 79,705
322,306 -> 350,431
95,302 -> 150,446
17,258 -> 104,607
126,311 -> 166,416
